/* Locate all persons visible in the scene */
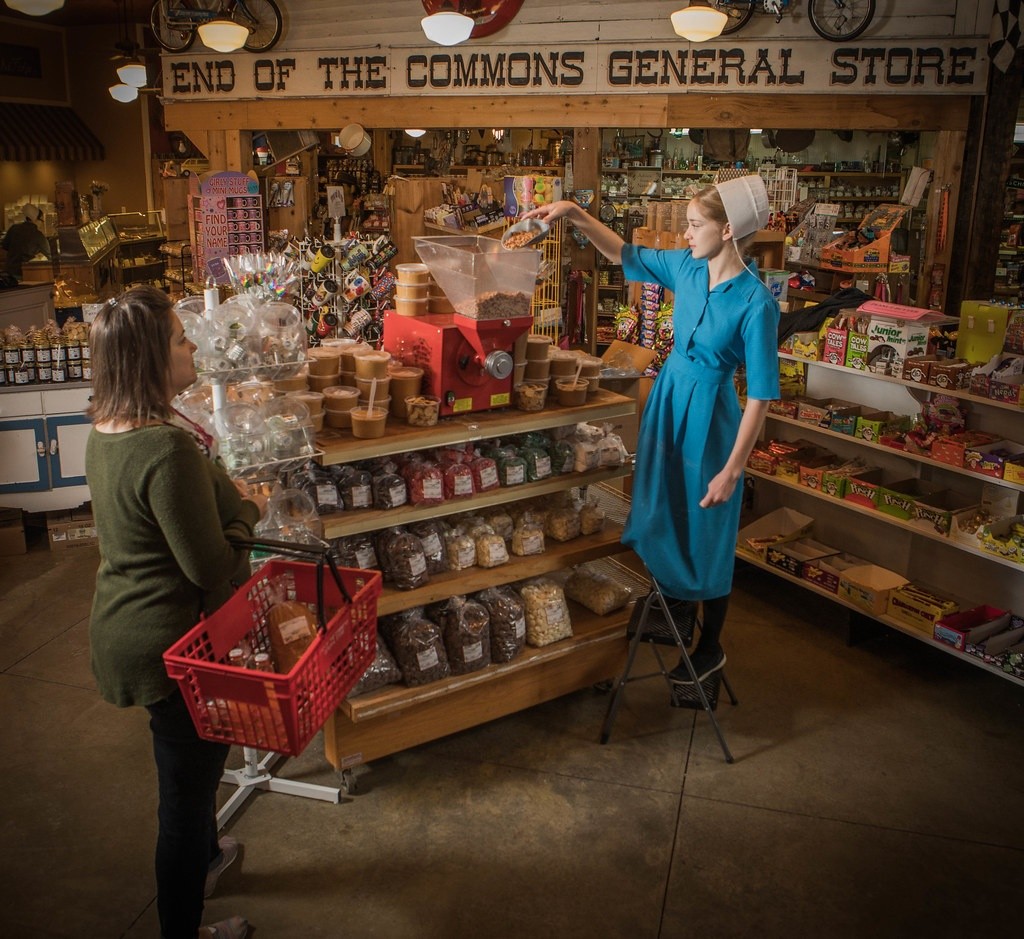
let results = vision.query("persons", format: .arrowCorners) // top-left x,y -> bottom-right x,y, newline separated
520,175 -> 780,685
2,209 -> 50,281
324,172 -> 361,239
87,287 -> 268,939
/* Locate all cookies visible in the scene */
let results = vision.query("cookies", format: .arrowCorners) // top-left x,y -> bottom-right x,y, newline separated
66,322 -> 91,339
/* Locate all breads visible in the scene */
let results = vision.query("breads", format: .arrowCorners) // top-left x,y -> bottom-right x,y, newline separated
266,600 -> 318,675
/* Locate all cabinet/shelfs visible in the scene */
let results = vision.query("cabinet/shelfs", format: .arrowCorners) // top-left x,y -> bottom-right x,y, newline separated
596,157 -> 937,346
1,145 -> 646,796
736,301 -> 1024,692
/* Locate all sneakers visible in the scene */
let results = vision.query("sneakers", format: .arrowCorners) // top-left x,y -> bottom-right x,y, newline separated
669,644 -> 726,684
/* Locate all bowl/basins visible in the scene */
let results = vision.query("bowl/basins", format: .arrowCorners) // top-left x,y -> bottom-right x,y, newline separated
271,338 -> 441,438
393,262 -> 456,317
513,330 -> 603,411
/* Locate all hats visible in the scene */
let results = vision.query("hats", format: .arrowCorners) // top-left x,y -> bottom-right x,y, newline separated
715,173 -> 770,241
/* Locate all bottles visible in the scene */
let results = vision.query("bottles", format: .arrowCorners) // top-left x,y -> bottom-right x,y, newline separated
229,649 -> 274,675
395,127 -> 900,197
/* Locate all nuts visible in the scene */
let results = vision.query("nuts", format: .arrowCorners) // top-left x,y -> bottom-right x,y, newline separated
441,495 -> 632,646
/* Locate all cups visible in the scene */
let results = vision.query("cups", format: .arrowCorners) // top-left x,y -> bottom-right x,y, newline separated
227,198 -> 261,256
280,234 -> 399,338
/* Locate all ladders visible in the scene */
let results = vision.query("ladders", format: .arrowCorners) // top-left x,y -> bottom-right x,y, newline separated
599,575 -> 738,763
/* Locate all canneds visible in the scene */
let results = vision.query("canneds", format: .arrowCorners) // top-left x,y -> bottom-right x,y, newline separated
0,340 -> 92,386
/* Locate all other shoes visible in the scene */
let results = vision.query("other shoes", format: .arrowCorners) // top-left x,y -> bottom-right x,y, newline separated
198,916 -> 248,939
201,835 -> 237,898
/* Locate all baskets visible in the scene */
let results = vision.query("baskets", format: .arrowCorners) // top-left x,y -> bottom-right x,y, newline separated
163,541 -> 382,755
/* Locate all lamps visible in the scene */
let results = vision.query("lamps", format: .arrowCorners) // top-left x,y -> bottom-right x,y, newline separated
670,0 -> 728,42
117,50 -> 147,88
109,83 -> 139,103
197,0 -> 249,53
421,0 -> 475,46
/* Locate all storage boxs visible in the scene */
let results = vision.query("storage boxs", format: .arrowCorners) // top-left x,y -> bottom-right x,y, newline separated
739,304 -> 1024,680
163,537 -> 383,755
